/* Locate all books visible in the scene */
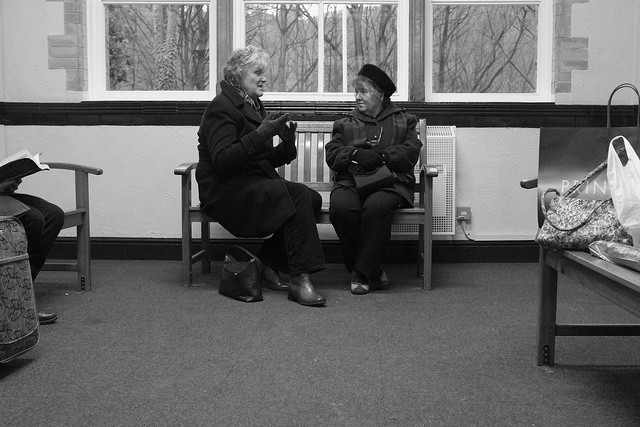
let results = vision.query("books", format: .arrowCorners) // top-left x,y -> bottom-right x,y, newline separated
0,149 -> 51,184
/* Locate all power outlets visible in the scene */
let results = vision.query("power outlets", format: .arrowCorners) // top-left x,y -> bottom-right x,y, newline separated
457,206 -> 471,221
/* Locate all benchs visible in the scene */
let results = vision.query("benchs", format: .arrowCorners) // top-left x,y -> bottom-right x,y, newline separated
520,175 -> 640,366
16,160 -> 103,293
173,119 -> 438,291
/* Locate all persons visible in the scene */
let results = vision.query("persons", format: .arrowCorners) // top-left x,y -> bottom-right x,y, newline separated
0,175 -> 65,326
192,42 -> 329,307
325,64 -> 422,295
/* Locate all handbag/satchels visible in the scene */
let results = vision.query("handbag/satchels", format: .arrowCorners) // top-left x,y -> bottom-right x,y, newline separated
219,244 -> 265,303
534,188 -> 631,251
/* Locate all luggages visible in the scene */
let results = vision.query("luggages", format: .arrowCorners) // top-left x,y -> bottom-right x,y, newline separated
0,207 -> 42,376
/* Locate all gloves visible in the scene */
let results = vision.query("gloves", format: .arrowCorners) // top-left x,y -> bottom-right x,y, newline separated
256,111 -> 293,143
282,120 -> 298,147
351,145 -> 383,170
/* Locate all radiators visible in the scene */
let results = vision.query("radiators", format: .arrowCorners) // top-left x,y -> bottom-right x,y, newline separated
391,122 -> 455,235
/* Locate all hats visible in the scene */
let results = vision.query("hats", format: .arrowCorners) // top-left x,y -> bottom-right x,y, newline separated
358,63 -> 396,97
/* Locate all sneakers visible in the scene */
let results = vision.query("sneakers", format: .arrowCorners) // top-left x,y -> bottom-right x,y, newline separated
375,265 -> 390,289
350,264 -> 371,295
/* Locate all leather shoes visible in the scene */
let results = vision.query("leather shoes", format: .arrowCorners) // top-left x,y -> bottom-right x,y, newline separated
36,307 -> 57,331
265,266 -> 289,291
287,273 -> 326,307
